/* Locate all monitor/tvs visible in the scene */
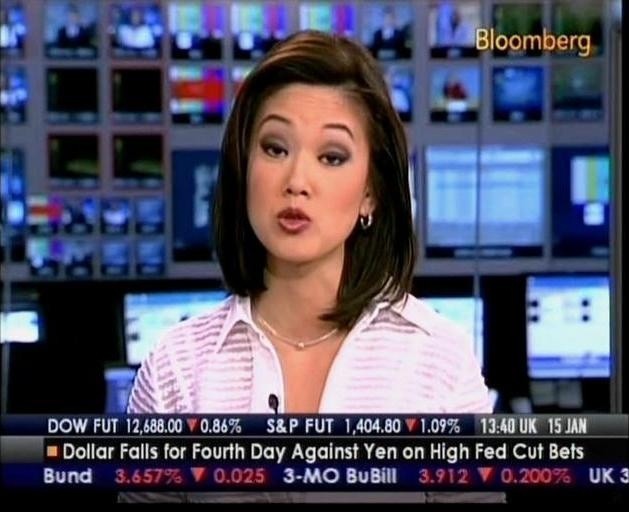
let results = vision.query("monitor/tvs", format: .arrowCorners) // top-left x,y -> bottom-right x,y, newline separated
411,291 -> 489,384
100,363 -> 141,414
0,0 -> 611,281
520,272 -> 611,383
120,286 -> 233,368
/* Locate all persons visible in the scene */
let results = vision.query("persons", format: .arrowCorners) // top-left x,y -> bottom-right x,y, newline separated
364,9 -> 412,60
55,4 -> 89,48
123,27 -> 509,505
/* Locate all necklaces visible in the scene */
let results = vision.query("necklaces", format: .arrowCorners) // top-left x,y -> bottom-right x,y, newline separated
255,310 -> 337,350
